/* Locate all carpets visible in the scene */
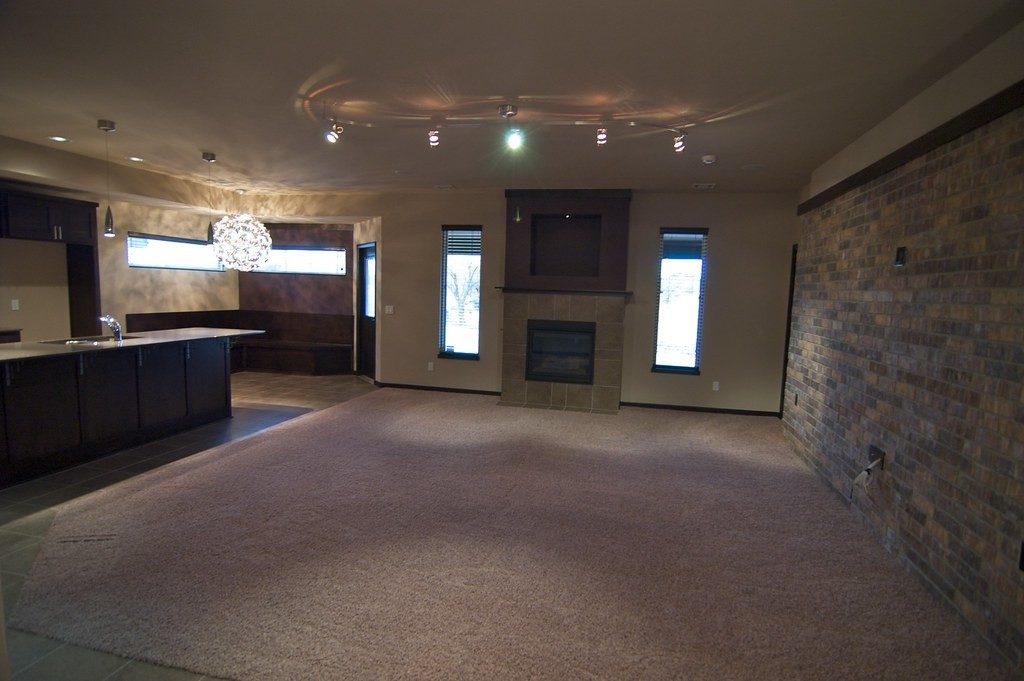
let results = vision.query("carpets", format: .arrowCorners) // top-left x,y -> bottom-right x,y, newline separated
6,385 -> 1024,681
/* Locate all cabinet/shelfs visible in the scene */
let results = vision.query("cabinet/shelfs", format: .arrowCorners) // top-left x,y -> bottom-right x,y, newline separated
0,187 -> 99,247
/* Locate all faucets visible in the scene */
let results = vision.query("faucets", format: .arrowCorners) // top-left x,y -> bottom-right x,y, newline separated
98,314 -> 122,341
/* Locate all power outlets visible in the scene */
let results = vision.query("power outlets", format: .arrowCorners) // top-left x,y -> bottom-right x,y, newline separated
894,246 -> 907,267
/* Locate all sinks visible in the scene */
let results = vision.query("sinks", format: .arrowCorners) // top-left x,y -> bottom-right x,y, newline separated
38,338 -> 99,348
89,336 -> 145,342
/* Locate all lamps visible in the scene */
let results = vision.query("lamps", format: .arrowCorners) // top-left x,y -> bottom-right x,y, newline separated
213,188 -> 273,272
426,115 -> 442,153
672,119 -> 687,153
323,103 -> 345,145
596,119 -> 608,149
202,152 -> 217,245
97,119 -> 117,239
